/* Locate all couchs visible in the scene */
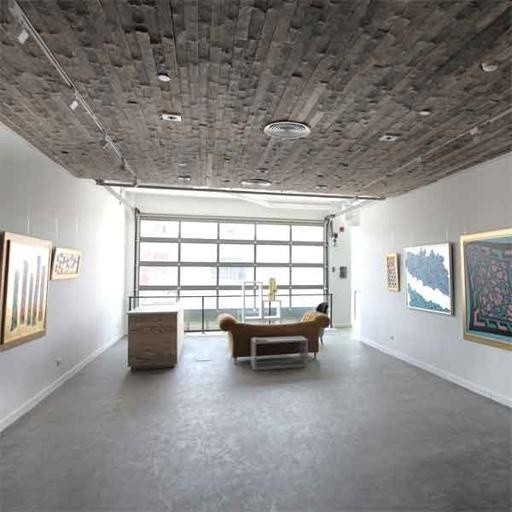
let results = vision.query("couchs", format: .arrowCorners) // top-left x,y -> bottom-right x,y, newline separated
217,311 -> 330,366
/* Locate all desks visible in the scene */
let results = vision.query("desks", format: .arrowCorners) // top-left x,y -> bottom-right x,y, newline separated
249,335 -> 308,371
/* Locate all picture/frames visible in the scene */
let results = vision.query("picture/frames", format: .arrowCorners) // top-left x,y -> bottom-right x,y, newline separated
385,227 -> 512,351
0,231 -> 82,352
